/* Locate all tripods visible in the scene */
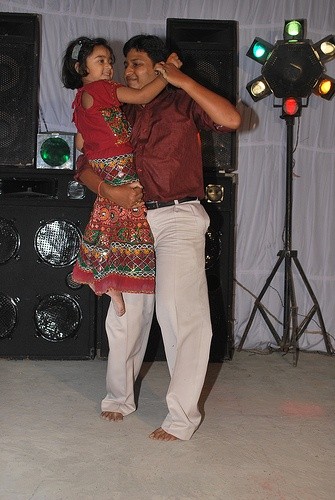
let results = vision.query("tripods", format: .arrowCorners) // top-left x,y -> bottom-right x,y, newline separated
237,115 -> 334,366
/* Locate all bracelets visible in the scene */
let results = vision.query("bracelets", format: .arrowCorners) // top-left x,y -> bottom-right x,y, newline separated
98,180 -> 105,198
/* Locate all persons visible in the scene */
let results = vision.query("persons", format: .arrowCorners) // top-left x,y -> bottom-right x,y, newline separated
62,37 -> 182,316
74,34 -> 241,441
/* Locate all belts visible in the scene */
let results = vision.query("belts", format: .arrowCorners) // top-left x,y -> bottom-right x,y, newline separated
144,197 -> 197,210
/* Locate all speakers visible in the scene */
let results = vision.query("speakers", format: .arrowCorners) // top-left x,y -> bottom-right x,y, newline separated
0,11 -> 239,362
